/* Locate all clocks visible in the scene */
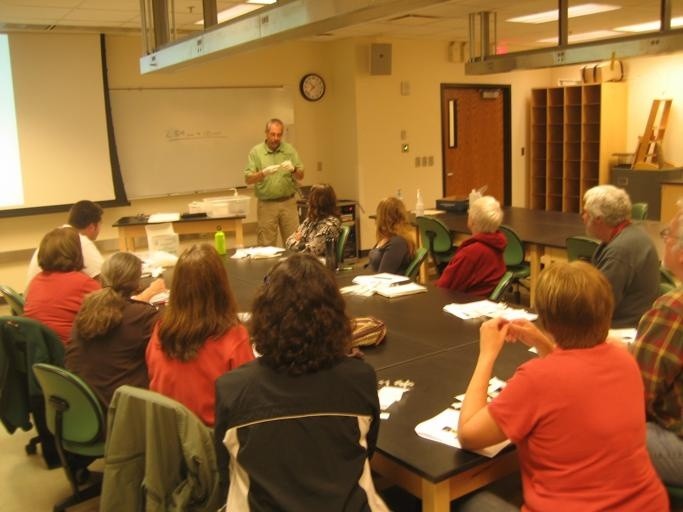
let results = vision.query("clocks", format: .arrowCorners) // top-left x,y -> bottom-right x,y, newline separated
300,72 -> 327,103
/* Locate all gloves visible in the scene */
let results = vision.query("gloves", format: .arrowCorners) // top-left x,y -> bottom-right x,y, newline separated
262,165 -> 281,177
281,160 -> 296,172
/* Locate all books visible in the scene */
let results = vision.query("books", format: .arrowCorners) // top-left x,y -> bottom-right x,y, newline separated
338,269 -> 428,302
438,293 -> 538,328
411,392 -> 513,460
226,242 -> 289,258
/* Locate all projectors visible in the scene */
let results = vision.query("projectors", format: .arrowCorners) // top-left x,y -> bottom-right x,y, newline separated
189,194 -> 250,217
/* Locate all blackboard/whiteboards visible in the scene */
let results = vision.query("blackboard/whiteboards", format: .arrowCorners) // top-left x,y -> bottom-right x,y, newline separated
106,85 -> 296,202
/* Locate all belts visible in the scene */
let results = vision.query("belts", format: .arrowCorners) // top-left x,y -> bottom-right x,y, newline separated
258,192 -> 295,203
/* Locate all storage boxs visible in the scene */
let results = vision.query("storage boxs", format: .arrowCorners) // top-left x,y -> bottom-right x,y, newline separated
607,164 -> 682,222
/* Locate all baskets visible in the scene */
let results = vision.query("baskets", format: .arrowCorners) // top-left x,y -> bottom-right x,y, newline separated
202,195 -> 251,218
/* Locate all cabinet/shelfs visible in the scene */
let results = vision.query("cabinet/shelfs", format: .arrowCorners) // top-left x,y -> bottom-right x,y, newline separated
527,80 -> 632,216
658,180 -> 682,222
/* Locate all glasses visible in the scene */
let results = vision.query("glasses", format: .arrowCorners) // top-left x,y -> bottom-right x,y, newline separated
659,228 -> 680,240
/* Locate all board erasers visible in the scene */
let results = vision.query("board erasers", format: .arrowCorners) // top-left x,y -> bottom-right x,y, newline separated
235,185 -> 247,189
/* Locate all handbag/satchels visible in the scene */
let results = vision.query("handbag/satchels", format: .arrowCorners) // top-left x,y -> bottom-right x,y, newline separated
350,317 -> 387,347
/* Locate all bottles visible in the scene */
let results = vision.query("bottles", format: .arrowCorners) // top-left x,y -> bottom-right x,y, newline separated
214,225 -> 225,255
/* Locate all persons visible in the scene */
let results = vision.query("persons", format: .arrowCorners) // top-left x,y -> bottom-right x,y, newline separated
20,229 -> 100,344
243,117 -> 306,249
142,245 -> 253,428
366,196 -> 414,276
629,200 -> 683,481
430,193 -> 507,293
286,183 -> 342,257
61,251 -> 159,409
213,252 -> 393,512
23,198 -> 138,343
457,260 -> 671,511
581,183 -> 660,328
